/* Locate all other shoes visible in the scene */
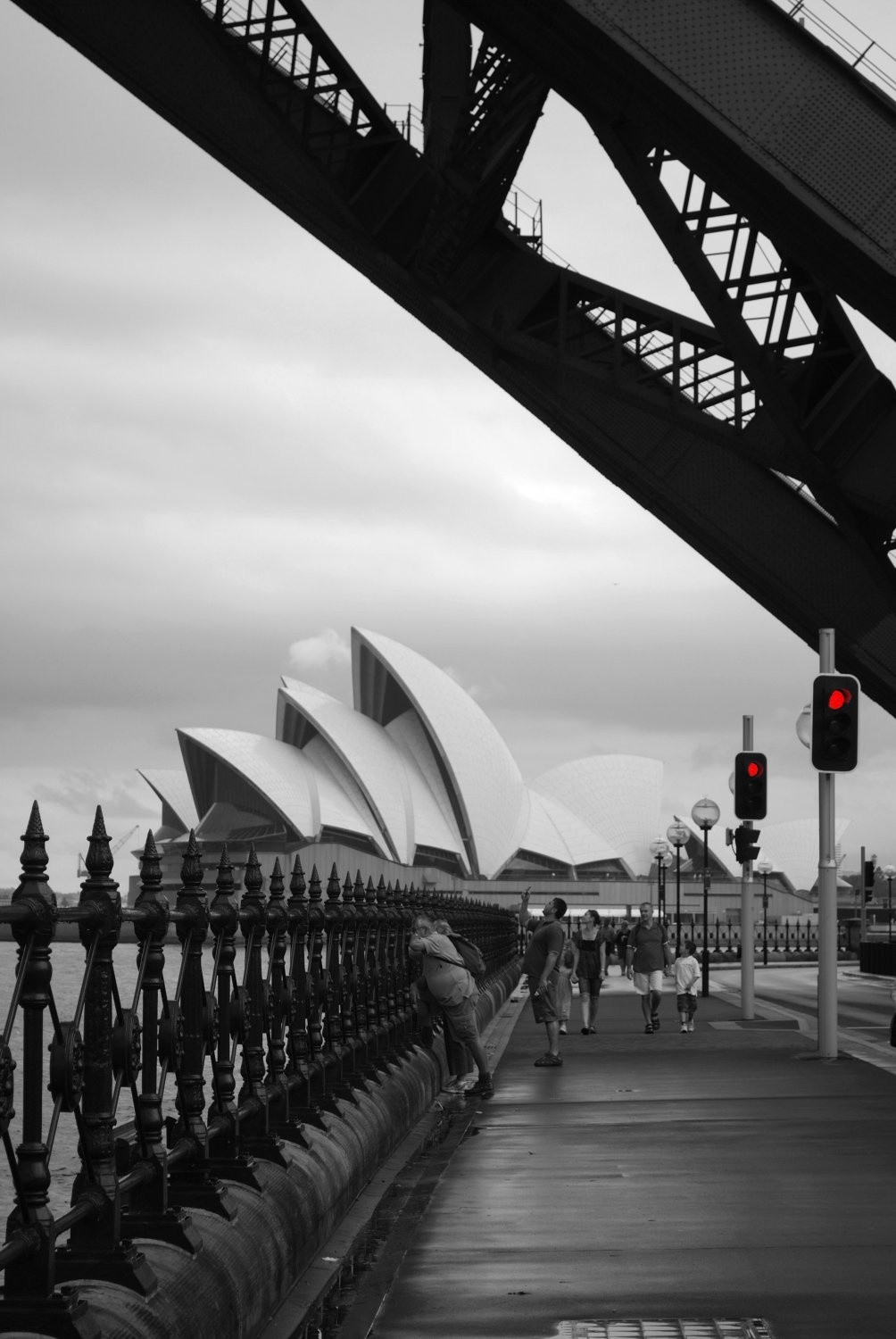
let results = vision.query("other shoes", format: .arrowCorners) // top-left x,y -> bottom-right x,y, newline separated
681,1025 -> 688,1032
581,1028 -> 590,1035
689,1024 -> 694,1031
560,1024 -> 568,1034
590,1025 -> 597,1034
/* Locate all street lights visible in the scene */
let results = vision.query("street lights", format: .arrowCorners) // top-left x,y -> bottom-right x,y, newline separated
649,837 -> 673,967
665,821 -> 691,959
691,798 -> 721,998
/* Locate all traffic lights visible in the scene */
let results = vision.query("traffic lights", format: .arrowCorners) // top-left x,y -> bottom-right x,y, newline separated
735,826 -> 761,863
733,751 -> 768,820
810,674 -> 860,773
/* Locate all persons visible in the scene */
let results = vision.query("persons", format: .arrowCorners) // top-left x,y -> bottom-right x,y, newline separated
667,940 -> 701,1031
572,910 -> 606,1034
434,920 -> 474,1092
555,935 -> 580,1033
520,886 -> 567,1067
615,920 -> 633,976
562,930 -> 578,977
600,918 -> 615,976
627,902 -> 671,1034
409,917 -> 492,1094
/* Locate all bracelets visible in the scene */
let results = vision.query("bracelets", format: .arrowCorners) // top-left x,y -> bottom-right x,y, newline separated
601,968 -> 605,971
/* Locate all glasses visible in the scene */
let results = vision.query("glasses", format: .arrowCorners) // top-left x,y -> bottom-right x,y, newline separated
414,926 -> 426,933
641,910 -> 652,913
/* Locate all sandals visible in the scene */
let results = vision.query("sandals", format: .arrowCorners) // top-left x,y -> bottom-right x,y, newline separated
651,1012 -> 661,1031
645,1024 -> 654,1034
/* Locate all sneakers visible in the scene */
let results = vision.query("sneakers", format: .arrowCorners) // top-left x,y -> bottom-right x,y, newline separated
464,1081 -> 494,1099
533,1050 -> 563,1067
441,1079 -> 468,1093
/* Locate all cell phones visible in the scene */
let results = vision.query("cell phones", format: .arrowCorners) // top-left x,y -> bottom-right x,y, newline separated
526,886 -> 531,895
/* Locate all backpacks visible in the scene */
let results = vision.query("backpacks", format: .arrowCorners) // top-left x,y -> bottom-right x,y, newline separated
427,935 -> 486,979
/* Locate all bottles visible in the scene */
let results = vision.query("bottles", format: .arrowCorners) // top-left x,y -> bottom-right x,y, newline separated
534,979 -> 553,996
